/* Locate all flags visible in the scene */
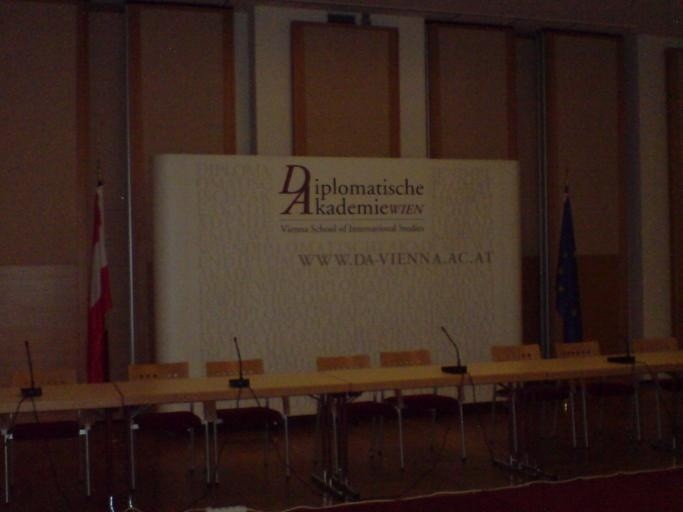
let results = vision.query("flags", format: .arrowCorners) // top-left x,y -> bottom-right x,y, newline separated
82,184 -> 112,383
553,184 -> 586,344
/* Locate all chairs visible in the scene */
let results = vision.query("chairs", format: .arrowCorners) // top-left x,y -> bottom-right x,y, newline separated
0,335 -> 679,503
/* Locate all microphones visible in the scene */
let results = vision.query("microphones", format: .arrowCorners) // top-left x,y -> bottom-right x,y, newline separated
229,336 -> 249,387
603,320 -> 635,364
440,326 -> 467,374
21,340 -> 43,397
